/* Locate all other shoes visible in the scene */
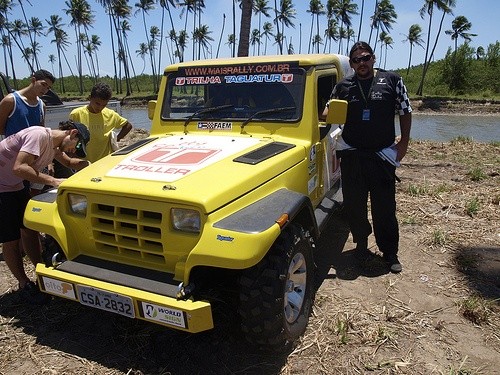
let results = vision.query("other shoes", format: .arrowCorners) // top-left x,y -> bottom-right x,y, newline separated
383,253 -> 402,272
19,280 -> 50,304
355,238 -> 367,261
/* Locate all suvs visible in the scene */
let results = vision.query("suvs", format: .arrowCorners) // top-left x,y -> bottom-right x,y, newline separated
21,52 -> 355,355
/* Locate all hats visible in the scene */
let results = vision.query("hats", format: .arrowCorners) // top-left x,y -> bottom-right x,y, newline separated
70,122 -> 90,158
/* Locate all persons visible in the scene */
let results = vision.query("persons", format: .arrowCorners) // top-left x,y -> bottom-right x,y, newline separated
0,121 -> 90,303
318,40 -> 412,272
69,82 -> 132,164
0,69 -> 55,138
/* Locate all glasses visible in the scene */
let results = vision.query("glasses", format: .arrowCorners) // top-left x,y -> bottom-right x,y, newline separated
75,132 -> 83,158
350,53 -> 372,64
